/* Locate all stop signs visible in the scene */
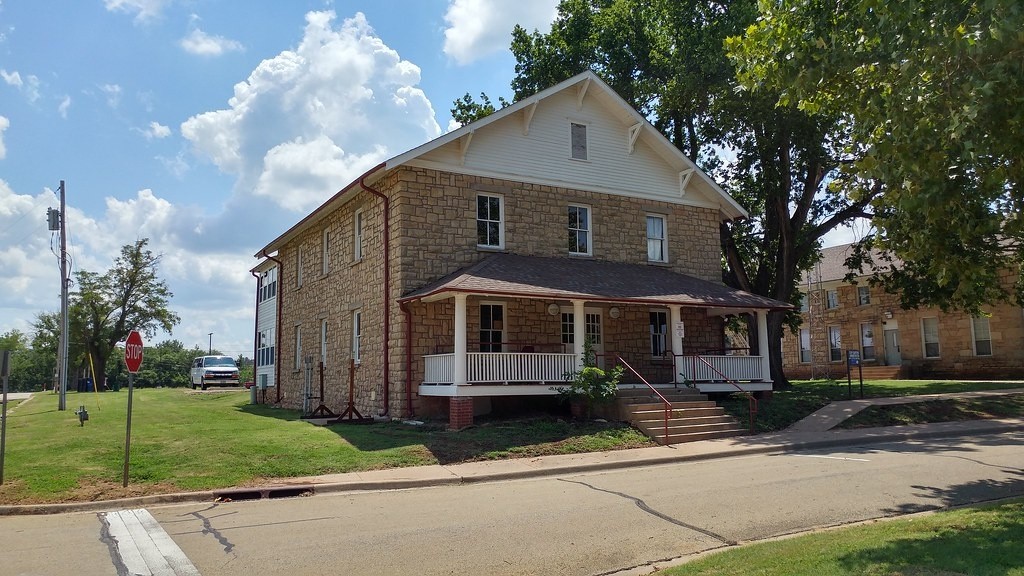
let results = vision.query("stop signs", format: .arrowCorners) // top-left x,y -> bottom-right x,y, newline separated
124,330 -> 143,373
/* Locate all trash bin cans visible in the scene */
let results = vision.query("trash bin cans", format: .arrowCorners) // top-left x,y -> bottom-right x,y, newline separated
249,386 -> 258,405
82,378 -> 93,392
78,378 -> 86,392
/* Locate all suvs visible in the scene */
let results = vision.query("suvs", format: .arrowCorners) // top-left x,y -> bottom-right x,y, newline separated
191,355 -> 240,390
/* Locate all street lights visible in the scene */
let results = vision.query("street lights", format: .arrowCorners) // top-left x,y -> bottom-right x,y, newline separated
208,332 -> 213,355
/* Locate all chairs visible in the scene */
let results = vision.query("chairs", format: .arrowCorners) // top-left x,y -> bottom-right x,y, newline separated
501,346 -> 534,385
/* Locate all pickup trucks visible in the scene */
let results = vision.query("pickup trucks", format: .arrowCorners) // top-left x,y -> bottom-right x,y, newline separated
245,382 -> 255,389
